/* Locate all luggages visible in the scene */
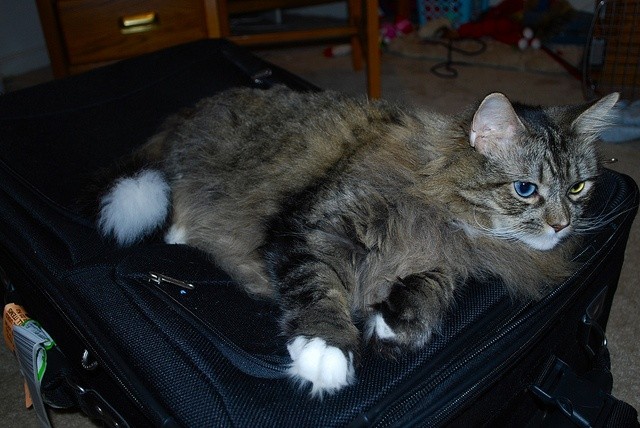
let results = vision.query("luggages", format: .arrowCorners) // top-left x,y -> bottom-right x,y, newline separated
1,37 -> 640,428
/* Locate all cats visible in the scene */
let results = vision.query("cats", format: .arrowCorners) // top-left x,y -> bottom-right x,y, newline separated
97,87 -> 619,402
